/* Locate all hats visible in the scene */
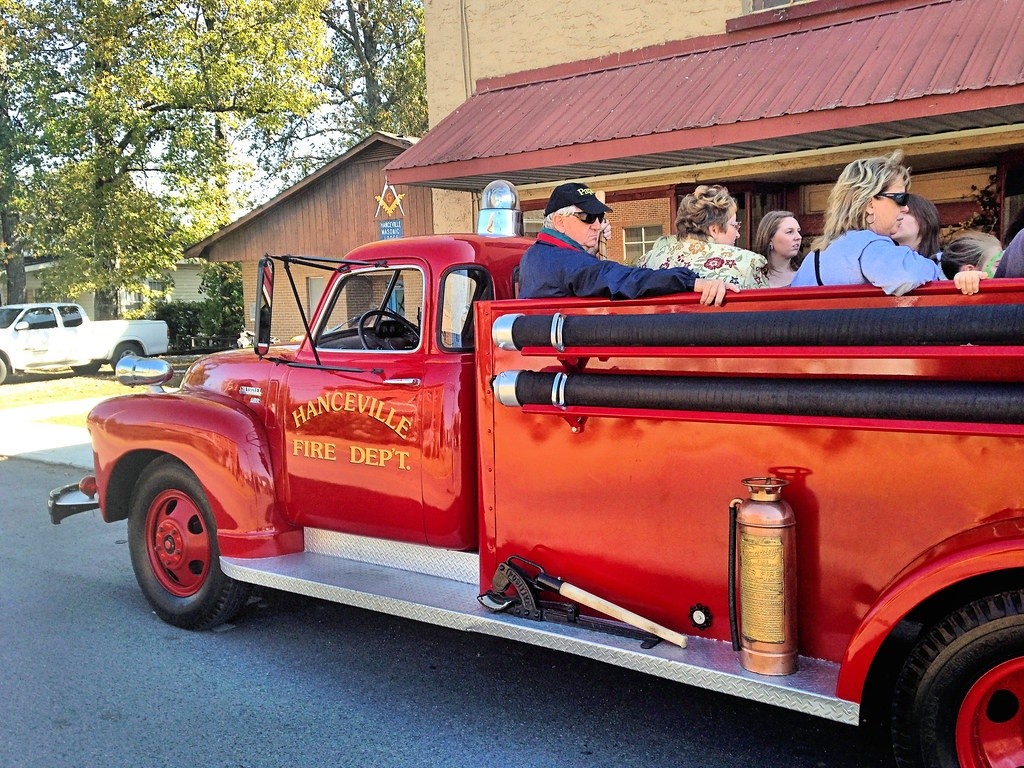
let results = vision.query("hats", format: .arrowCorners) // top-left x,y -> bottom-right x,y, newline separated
544,182 -> 613,218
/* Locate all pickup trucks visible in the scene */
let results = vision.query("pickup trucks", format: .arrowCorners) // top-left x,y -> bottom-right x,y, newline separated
1,301 -> 171,384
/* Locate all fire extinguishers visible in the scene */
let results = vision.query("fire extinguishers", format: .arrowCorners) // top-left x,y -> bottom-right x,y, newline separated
728,477 -> 796,676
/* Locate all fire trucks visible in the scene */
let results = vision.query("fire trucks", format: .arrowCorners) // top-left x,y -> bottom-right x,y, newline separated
41,176 -> 1024,766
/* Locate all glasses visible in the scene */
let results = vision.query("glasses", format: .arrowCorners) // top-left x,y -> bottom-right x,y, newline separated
570,212 -> 606,224
727,220 -> 742,231
880,192 -> 911,207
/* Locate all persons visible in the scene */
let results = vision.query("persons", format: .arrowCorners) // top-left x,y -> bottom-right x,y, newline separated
754,210 -> 806,288
1004,209 -> 1023,247
517,182 -> 742,307
790,149 -> 944,298
892,194 -> 950,281
940,228 -> 1004,296
993,226 -> 1024,279
628,186 -> 771,293
